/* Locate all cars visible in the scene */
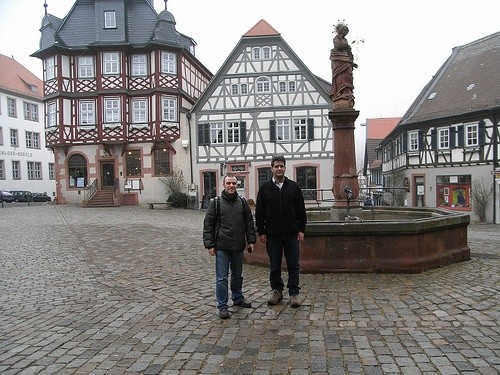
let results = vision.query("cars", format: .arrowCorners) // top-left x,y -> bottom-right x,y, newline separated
0,190 -> 14,204
32,193 -> 51,202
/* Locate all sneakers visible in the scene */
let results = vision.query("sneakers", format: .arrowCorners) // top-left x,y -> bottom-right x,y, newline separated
290,293 -> 300,307
268,289 -> 283,305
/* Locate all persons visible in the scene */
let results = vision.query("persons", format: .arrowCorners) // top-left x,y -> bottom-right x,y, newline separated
329,24 -> 359,110
255,155 -> 307,308
203,175 -> 257,319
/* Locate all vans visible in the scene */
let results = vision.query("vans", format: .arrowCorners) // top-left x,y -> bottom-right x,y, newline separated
10,191 -> 32,203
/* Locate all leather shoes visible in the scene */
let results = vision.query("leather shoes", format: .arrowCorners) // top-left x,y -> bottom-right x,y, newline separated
219,308 -> 229,318
232,296 -> 251,308
